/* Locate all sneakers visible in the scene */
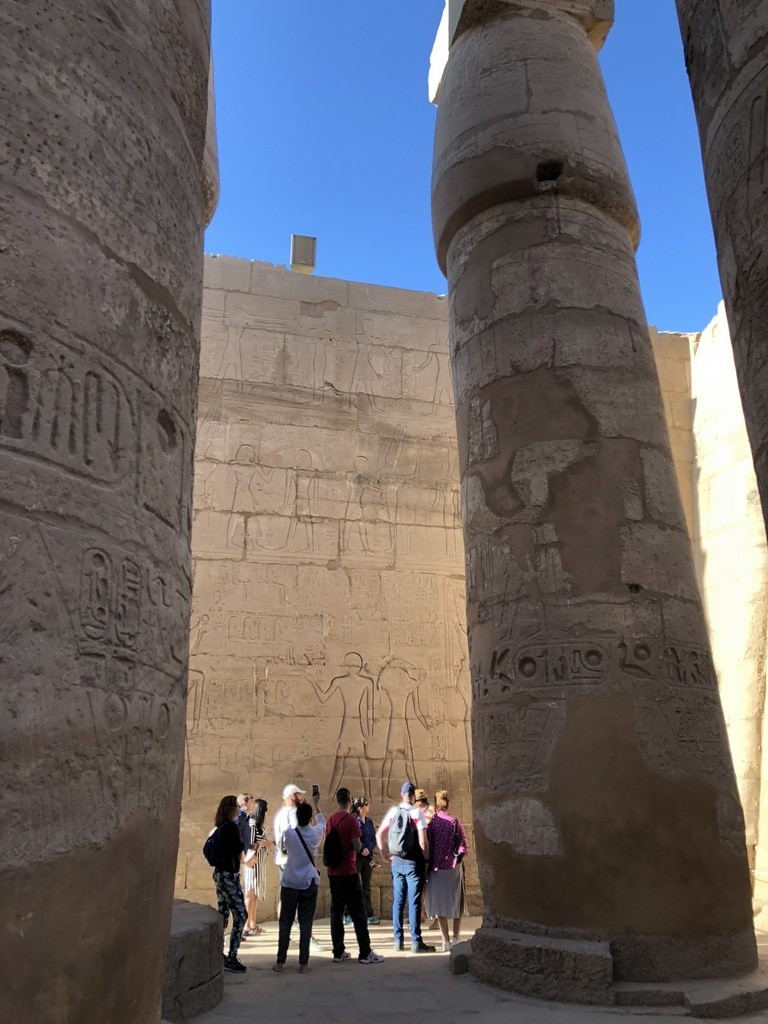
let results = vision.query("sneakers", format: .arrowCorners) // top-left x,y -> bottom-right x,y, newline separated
359,949 -> 384,963
333,951 -> 351,962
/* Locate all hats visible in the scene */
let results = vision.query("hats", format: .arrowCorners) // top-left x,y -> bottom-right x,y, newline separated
415,789 -> 427,800
401,782 -> 417,793
282,784 -> 306,798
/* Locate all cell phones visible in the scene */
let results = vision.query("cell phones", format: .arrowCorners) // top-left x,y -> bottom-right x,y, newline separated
312,784 -> 319,796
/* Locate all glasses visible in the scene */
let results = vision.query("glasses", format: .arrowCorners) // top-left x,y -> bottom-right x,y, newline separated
421,799 -> 427,803
250,796 -> 253,799
362,803 -> 368,806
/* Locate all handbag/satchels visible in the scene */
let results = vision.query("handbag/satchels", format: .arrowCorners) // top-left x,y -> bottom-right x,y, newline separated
454,818 -> 464,863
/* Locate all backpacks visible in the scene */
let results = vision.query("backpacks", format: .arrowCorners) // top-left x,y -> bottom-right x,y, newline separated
388,807 -> 414,855
323,814 -> 351,867
203,822 -> 227,868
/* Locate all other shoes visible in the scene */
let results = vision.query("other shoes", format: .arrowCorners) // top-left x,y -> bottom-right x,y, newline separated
272,963 -> 283,972
224,954 -> 247,973
411,941 -> 436,952
344,915 -> 353,924
442,942 -> 451,952
223,953 -> 227,963
395,940 -> 404,951
243,927 -> 264,936
367,916 -> 380,925
299,964 -> 311,973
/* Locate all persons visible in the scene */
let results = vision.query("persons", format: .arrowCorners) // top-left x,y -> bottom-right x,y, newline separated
344,796 -> 381,925
272,784 -> 325,950
203,794 -> 273,973
322,787 -> 385,963
375,781 -> 469,953
272,792 -> 327,975
